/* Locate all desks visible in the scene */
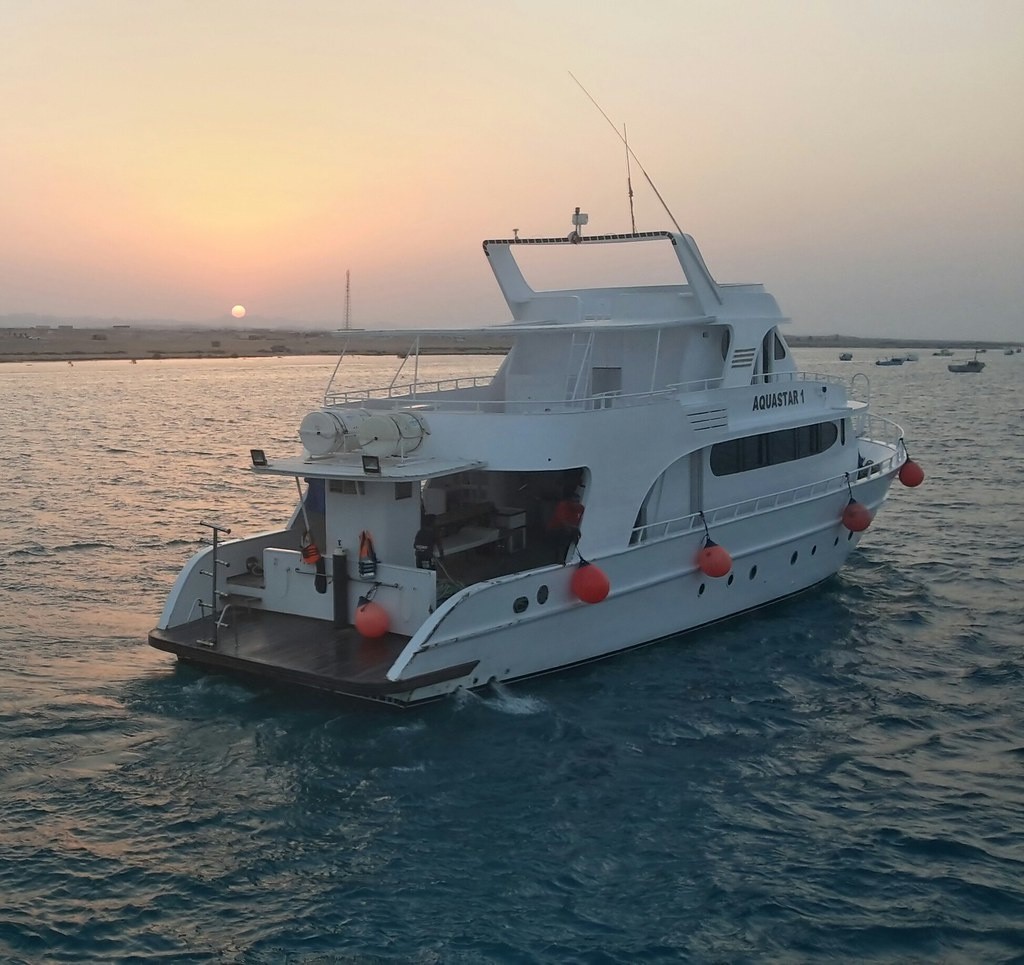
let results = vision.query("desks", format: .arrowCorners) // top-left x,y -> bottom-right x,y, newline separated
506,526 -> 526,553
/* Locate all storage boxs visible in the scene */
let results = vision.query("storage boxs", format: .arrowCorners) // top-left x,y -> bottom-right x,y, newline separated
496,506 -> 527,529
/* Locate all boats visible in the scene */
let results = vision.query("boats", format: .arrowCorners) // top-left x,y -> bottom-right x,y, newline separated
932,348 -> 954,356
904,350 -> 917,360
146,69 -> 927,714
839,352 -> 853,361
947,344 -> 1022,374
875,355 -> 905,366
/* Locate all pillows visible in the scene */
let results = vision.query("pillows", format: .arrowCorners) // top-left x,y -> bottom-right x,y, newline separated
459,526 -> 500,538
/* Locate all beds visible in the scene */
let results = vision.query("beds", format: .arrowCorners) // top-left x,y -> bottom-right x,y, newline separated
433,525 -> 515,558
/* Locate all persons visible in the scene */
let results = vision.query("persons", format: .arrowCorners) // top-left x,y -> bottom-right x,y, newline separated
414,514 -> 444,570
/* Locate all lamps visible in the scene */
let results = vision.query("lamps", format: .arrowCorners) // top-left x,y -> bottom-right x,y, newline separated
362,456 -> 381,473
250,449 -> 267,466
